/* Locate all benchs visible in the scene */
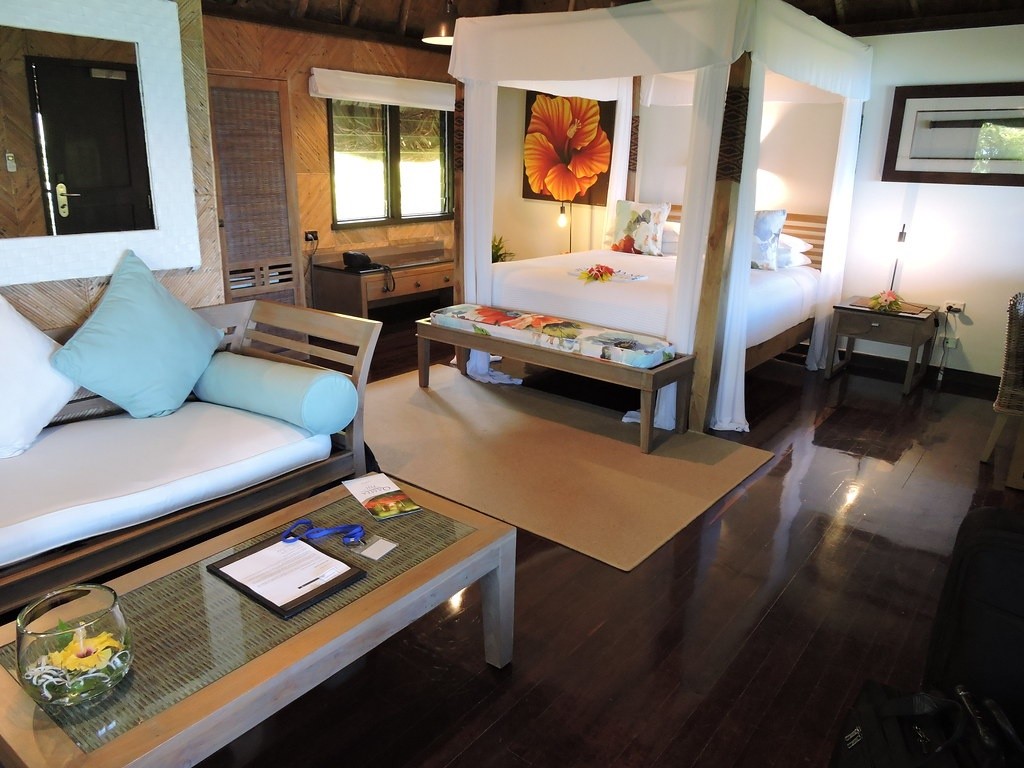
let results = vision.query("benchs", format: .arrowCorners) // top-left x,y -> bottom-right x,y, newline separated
415,303 -> 696,455
0,300 -> 384,614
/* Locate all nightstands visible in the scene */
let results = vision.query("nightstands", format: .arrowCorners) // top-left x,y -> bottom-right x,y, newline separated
824,295 -> 940,395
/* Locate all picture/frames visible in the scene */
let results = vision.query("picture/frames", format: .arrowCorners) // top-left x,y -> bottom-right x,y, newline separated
882,80 -> 1024,188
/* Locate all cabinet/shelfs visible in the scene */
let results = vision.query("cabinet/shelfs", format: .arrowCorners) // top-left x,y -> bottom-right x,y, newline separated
311,240 -> 456,385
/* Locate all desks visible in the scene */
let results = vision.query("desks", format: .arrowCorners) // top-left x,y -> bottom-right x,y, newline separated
0,471 -> 519,768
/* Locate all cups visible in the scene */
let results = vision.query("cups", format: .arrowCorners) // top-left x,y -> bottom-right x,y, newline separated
15,584 -> 134,705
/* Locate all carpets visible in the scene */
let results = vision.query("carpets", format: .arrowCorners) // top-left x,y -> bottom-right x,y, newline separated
363,363 -> 774,572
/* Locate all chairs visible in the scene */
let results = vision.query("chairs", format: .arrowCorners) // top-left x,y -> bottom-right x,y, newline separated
981,291 -> 1024,490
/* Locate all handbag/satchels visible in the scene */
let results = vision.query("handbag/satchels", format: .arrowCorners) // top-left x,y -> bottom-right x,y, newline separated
342,250 -> 374,269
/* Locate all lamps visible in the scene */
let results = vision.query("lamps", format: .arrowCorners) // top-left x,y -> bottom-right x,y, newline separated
422,0 -> 460,46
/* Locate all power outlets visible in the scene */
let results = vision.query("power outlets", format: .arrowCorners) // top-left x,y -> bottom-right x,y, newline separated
943,302 -> 965,313
305,230 -> 317,240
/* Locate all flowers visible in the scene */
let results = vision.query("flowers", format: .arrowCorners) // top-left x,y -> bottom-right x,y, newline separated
575,263 -> 615,286
454,200 -> 827,434
24,617 -> 133,704
869,290 -> 905,313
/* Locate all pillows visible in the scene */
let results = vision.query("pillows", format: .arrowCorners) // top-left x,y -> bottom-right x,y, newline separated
192,352 -> 359,435
0,295 -> 82,459
612,200 -> 680,257
49,250 -> 225,419
750,209 -> 813,271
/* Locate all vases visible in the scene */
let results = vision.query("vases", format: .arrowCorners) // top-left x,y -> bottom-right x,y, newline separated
15,583 -> 134,707
